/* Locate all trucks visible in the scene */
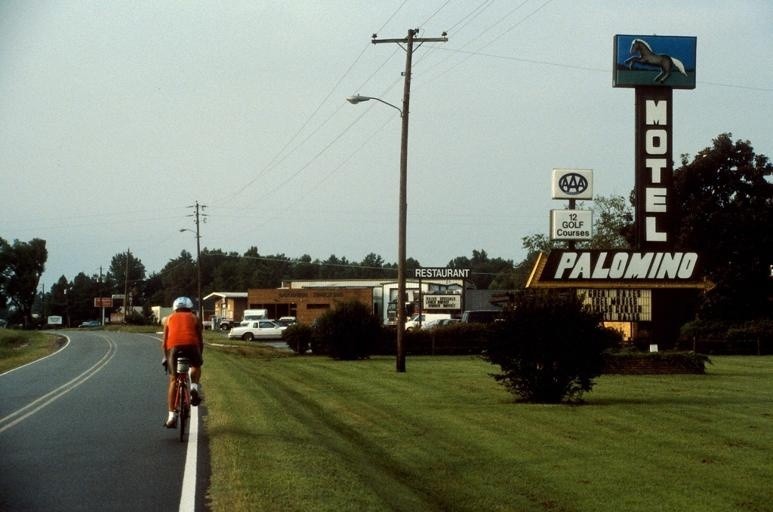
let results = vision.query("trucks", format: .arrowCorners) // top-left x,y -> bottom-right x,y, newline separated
404,312 -> 452,333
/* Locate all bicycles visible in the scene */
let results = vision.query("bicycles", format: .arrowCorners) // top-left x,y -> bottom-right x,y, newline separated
161,348 -> 193,442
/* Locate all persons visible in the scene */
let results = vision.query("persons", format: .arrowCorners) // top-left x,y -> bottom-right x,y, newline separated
160,295 -> 203,428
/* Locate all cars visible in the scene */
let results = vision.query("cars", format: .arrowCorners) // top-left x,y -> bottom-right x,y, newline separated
78,320 -> 102,328
227,319 -> 288,342
279,315 -> 296,326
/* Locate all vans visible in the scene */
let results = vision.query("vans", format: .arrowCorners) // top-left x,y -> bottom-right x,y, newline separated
241,308 -> 269,326
462,309 -> 505,325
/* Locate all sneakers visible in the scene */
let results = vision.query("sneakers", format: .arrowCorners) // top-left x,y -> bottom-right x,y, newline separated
190,389 -> 201,407
165,411 -> 177,428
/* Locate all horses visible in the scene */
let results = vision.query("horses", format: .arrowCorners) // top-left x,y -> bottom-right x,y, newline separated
623,38 -> 688,84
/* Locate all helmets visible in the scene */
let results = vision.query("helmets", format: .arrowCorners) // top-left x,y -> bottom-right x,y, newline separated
172,295 -> 193,311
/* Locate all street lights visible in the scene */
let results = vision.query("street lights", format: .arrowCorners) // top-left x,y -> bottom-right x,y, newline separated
180,201 -> 208,328
345,27 -> 449,370
